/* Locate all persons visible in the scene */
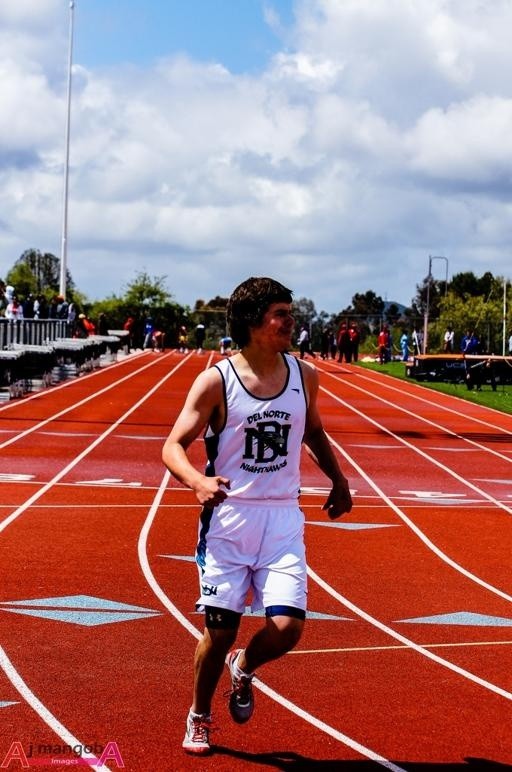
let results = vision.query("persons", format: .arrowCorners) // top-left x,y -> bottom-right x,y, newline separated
376,323 -> 424,365
296,319 -> 360,364
2,282 -> 95,338
178,322 -> 233,356
460,329 -> 478,354
98,312 -> 166,354
162,278 -> 356,754
443,326 -> 454,352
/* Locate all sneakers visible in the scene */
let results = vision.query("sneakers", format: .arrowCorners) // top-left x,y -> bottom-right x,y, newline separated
226,649 -> 254,724
183,706 -> 212,755
